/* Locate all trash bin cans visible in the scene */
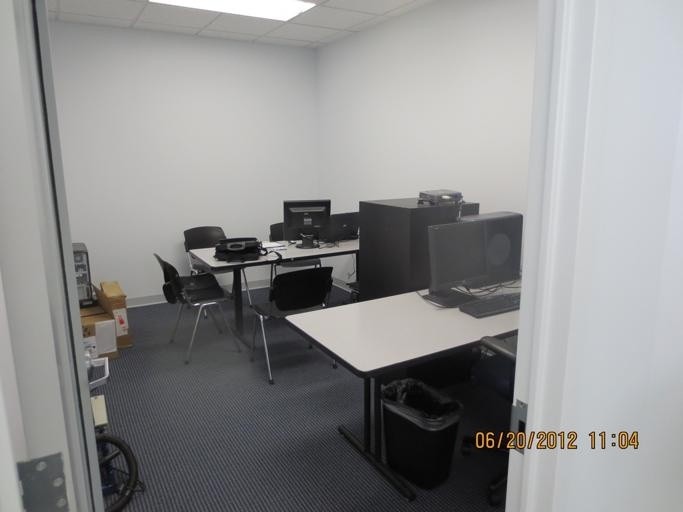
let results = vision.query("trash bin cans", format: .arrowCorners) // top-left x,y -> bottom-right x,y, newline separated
381,378 -> 463,491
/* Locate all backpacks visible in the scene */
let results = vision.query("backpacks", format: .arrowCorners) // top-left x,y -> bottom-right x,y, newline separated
213,237 -> 268,263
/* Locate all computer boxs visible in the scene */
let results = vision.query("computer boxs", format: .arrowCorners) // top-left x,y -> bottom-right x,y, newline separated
459,211 -> 522,289
72,242 -> 93,301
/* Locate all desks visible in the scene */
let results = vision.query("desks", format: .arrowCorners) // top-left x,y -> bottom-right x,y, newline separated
189,227 -> 359,351
284,266 -> 521,502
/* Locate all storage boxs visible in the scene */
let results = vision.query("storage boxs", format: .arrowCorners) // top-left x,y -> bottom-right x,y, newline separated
80,280 -> 134,359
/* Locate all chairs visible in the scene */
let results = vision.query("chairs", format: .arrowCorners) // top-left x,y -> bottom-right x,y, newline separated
153,253 -> 242,364
250,266 -> 337,385
269,222 -> 322,286
457,336 -> 516,502
184,226 -> 252,319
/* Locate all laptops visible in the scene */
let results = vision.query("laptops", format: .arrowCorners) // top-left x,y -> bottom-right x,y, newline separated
328,212 -> 359,243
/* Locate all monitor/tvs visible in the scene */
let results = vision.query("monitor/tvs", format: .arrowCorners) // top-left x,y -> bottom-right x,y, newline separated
424,220 -> 485,308
283,199 -> 330,249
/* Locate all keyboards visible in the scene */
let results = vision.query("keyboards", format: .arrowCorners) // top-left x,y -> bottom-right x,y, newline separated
460,292 -> 520,319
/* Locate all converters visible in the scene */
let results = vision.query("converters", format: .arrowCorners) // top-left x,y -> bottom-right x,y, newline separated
81,298 -> 95,307
